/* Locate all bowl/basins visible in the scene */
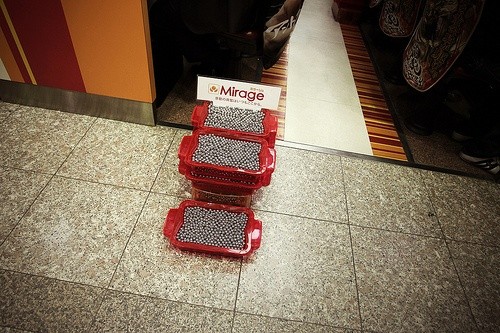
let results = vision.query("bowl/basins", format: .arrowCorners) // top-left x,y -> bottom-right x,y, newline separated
179,101 -> 279,205
162,199 -> 262,258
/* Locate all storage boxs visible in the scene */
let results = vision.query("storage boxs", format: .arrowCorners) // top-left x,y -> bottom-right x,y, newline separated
163,100 -> 277,257
